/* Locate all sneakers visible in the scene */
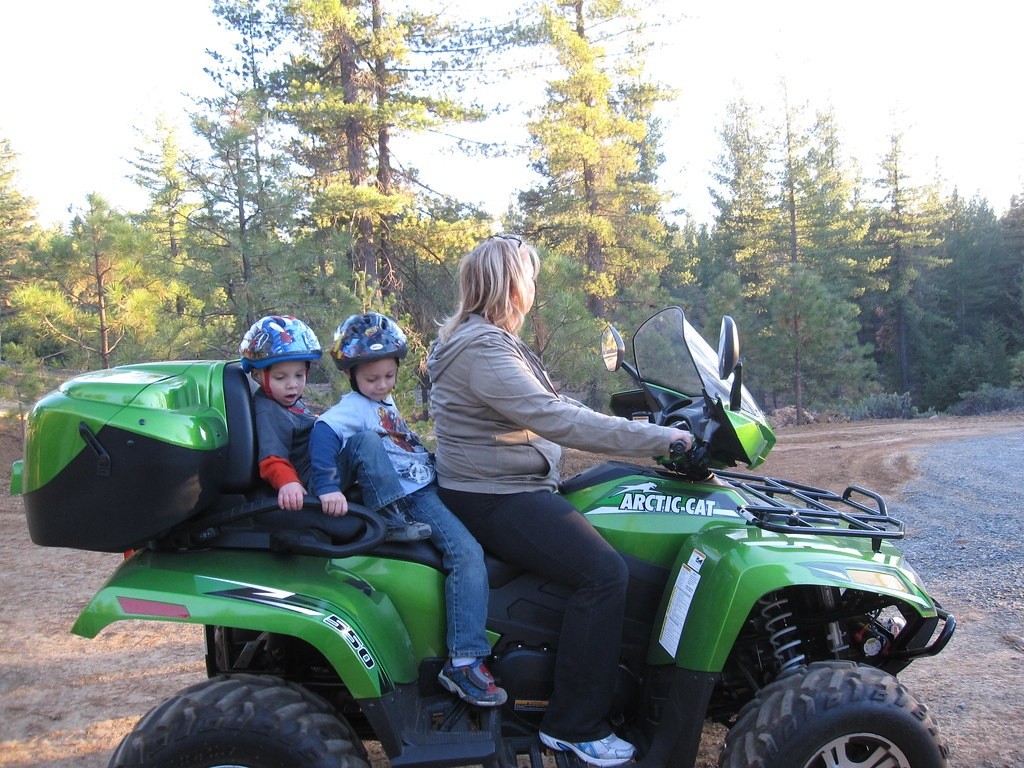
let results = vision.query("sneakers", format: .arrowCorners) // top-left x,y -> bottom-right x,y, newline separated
538,730 -> 636,766
438,656 -> 507,707
376,497 -> 432,543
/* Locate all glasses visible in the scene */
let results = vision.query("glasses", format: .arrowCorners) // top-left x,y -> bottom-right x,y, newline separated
487,235 -> 523,251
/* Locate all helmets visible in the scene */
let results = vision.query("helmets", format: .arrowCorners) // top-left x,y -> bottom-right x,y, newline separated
239,316 -> 322,374
330,312 -> 409,369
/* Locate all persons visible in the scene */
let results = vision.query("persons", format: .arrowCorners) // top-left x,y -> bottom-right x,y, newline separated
240,315 -> 432,543
426,232 -> 695,768
307,311 -> 508,708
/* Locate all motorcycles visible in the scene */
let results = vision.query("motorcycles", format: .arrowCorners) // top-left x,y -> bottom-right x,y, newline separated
9,306 -> 956,768
